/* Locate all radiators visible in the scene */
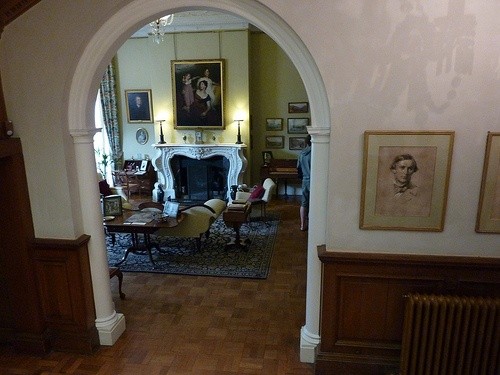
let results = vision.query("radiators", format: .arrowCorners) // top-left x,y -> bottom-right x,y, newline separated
399,293 -> 500,375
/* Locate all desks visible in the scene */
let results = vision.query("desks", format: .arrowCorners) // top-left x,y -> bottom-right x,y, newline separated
104,209 -> 182,267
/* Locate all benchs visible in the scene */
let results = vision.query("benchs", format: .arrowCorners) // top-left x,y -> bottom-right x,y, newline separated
137,198 -> 227,249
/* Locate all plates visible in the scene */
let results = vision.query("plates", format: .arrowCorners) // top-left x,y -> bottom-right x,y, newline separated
103,216 -> 115,221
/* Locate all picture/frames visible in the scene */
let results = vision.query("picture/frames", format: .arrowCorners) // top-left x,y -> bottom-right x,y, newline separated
170,58 -> 226,130
124,88 -> 155,125
103,195 -> 123,216
474,131 -> 500,234
359,130 -> 456,232
265,102 -> 309,150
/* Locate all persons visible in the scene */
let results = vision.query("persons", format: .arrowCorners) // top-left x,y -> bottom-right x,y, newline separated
296,134 -> 312,231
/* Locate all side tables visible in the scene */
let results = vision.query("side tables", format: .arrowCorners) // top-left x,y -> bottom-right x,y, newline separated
223,202 -> 252,243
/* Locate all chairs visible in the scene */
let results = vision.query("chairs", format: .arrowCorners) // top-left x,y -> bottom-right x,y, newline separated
233,178 -> 278,216
114,171 -> 138,199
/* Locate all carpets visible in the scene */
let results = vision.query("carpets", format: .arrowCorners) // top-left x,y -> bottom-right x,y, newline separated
104,213 -> 280,280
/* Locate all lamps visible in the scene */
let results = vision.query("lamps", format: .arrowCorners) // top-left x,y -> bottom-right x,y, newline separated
233,119 -> 246,145
149,15 -> 173,45
155,119 -> 167,144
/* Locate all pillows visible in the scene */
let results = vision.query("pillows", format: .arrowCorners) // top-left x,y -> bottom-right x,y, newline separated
247,187 -> 266,201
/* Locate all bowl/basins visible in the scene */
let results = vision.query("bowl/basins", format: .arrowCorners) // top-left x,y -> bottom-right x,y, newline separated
152,212 -> 169,223
